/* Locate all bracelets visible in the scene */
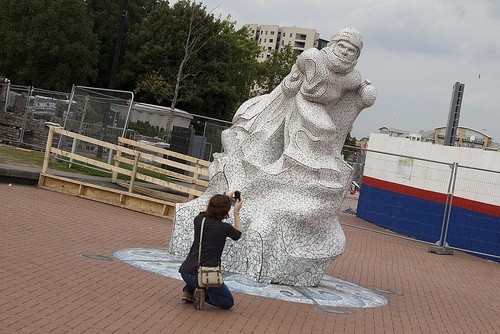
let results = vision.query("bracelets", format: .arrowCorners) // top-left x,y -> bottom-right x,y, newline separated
233,211 -> 240,215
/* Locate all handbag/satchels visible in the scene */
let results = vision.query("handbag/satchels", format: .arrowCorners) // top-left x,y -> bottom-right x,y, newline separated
197,266 -> 223,287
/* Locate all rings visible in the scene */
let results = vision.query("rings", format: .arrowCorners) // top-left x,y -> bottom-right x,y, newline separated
240,201 -> 242,203
178,190 -> 243,310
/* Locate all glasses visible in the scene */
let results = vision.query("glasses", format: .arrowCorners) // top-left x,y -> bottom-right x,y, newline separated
216,194 -> 229,203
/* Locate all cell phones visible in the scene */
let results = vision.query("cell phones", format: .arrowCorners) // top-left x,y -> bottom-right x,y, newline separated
235,191 -> 240,201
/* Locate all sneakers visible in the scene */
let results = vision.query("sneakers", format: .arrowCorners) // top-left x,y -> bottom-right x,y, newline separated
181,291 -> 196,302
194,288 -> 205,310
